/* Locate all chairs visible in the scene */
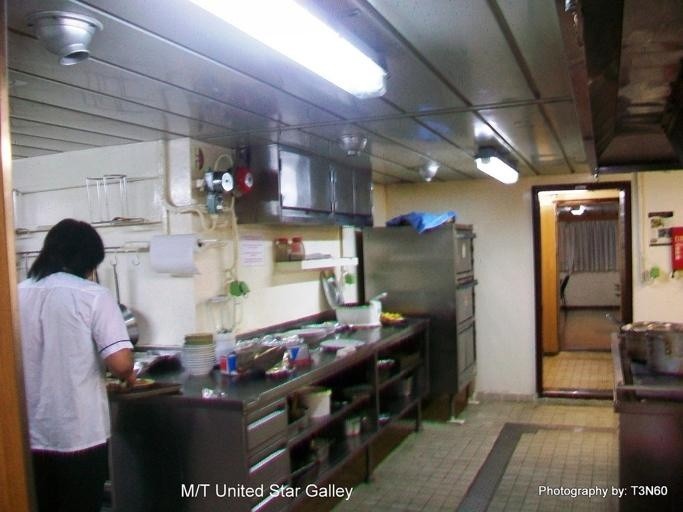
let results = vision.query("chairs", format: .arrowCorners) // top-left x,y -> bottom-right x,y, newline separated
559,275 -> 569,312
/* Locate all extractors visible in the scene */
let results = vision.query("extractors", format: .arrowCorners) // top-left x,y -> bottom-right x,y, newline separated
113,264 -> 139,345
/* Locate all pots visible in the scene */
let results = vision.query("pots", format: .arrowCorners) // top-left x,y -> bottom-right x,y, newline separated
605,313 -> 683,376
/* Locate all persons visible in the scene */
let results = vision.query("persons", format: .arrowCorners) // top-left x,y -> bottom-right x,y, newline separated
13,216 -> 137,512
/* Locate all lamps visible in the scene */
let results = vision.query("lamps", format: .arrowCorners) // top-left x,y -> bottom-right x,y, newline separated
475,147 -> 521,186
185,0 -> 390,101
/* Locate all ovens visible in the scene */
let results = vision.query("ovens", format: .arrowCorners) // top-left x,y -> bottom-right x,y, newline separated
362,224 -> 479,423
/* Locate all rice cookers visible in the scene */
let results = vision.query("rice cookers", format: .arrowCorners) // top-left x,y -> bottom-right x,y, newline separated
320,269 -> 388,328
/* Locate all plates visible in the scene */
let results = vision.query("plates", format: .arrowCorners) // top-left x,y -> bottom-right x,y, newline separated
105,377 -> 155,391
322,339 -> 366,352
288,327 -> 327,345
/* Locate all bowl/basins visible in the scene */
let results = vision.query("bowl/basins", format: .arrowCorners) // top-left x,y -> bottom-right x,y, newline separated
181,333 -> 216,375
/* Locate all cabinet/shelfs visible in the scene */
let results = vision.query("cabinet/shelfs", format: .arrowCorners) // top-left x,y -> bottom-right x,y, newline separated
234,144 -> 373,228
106,315 -> 430,511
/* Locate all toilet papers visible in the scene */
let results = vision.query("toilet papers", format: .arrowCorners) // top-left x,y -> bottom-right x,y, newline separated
149,233 -> 206,277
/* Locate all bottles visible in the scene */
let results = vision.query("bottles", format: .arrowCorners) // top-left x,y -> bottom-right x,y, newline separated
290,236 -> 306,260
275,238 -> 290,261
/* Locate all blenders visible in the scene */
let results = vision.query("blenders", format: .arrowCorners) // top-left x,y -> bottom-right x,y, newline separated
207,295 -> 245,364
